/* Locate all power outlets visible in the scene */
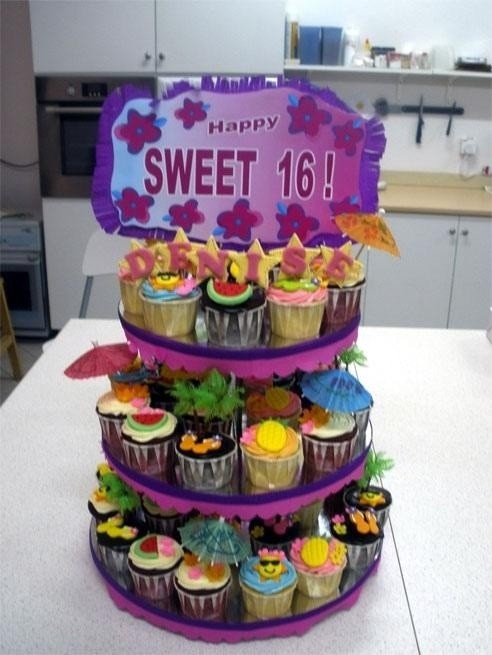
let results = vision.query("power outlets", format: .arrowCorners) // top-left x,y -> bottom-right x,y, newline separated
460,140 -> 476,155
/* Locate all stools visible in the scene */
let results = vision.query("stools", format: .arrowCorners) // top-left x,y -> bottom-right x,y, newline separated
0,278 -> 25,386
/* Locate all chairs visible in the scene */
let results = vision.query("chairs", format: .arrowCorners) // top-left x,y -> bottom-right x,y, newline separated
78,225 -> 142,319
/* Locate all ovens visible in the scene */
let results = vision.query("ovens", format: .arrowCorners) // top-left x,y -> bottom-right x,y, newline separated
35,76 -> 158,199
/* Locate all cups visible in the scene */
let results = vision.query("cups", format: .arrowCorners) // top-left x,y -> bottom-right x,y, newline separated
322,26 -> 342,67
299,26 -> 322,65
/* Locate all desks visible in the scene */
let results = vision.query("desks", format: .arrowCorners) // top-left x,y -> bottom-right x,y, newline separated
1,316 -> 492,652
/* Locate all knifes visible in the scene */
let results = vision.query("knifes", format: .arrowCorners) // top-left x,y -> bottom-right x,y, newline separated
416,95 -> 425,144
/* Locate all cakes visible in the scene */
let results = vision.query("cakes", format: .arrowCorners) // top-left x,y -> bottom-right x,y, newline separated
86,483 -> 138,524
239,416 -> 304,488
318,240 -> 366,323
96,512 -> 138,570
95,382 -> 150,449
289,533 -> 348,598
238,545 -> 300,618
172,553 -> 233,618
263,233 -> 333,339
127,531 -> 185,599
298,403 -> 359,471
243,387 -> 303,425
300,500 -> 324,528
139,226 -> 202,338
328,503 -> 385,568
248,514 -> 303,557
118,240 -> 154,314
140,495 -> 187,536
185,233 -> 279,349
344,452 -> 393,527
119,408 -> 180,477
174,429 -> 239,488
177,367 -> 245,432
356,398 -> 374,437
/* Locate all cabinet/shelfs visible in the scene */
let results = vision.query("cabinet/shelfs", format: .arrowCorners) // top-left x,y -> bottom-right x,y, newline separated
366,211 -> 492,333
42,195 -> 133,330
29,1 -> 286,80
